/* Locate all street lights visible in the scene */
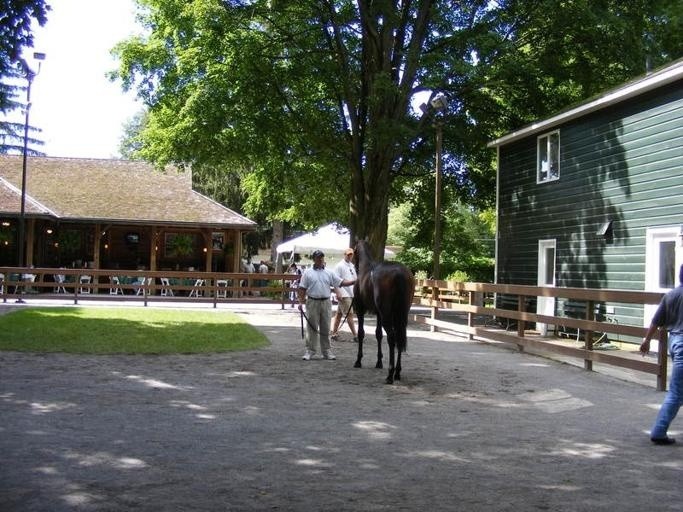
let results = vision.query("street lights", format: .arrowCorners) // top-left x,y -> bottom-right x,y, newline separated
6,49 -> 46,305
418,94 -> 449,318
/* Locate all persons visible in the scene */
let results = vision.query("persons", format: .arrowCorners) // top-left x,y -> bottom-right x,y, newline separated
637,261 -> 683,444
329,246 -> 361,343
295,248 -> 358,363
286,260 -> 302,308
256,258 -> 268,294
241,253 -> 256,296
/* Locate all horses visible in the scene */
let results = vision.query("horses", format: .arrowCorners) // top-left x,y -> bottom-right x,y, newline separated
352,235 -> 415,385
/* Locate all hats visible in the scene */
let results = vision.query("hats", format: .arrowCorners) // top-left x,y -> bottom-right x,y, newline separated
312,250 -> 324,260
345,248 -> 354,255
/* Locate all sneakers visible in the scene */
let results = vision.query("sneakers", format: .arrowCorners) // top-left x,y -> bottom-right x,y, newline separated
331,335 -> 346,342
651,437 -> 675,445
302,353 -> 310,361
322,350 -> 336,360
351,336 -> 359,343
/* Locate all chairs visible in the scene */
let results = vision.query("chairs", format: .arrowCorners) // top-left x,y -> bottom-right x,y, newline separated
0,274 -> 227,299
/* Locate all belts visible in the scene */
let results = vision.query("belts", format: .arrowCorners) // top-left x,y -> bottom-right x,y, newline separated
310,297 -> 328,300
